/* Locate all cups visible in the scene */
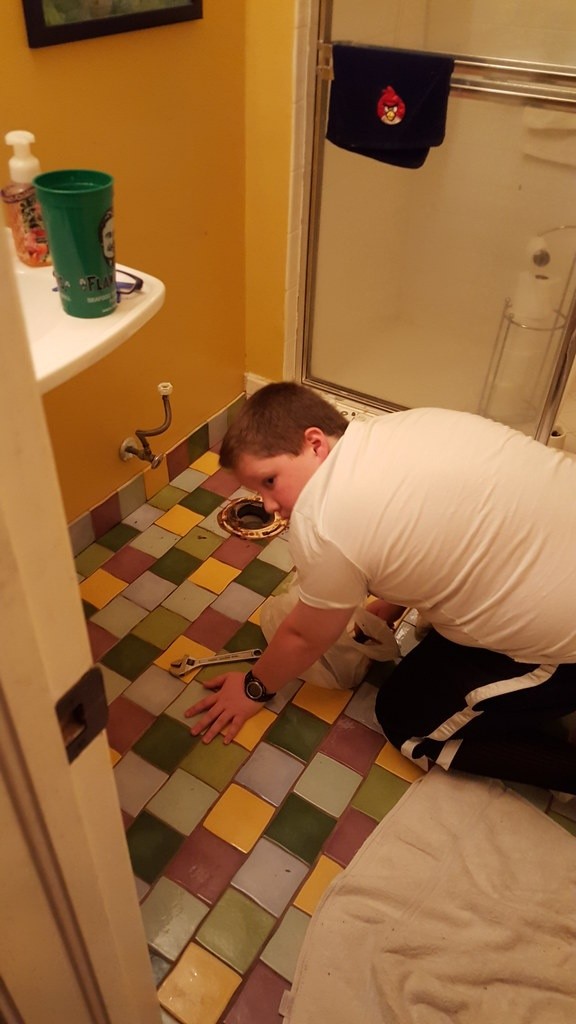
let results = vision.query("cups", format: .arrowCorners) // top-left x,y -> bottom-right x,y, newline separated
33,168 -> 118,318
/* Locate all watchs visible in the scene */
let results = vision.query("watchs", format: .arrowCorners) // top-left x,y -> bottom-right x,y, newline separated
243,669 -> 278,703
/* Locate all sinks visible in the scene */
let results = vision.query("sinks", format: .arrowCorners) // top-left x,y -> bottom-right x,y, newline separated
15,239 -> 167,393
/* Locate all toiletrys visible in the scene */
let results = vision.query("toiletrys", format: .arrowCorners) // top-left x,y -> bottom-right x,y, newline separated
5,130 -> 58,266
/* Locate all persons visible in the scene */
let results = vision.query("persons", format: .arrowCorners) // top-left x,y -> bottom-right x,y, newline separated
182,380 -> 576,800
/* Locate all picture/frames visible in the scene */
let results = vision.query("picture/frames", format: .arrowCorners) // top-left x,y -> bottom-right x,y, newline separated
21,0 -> 203,49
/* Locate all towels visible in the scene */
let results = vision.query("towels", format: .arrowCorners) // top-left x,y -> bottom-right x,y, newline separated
279,765 -> 576,1024
327,38 -> 456,169
